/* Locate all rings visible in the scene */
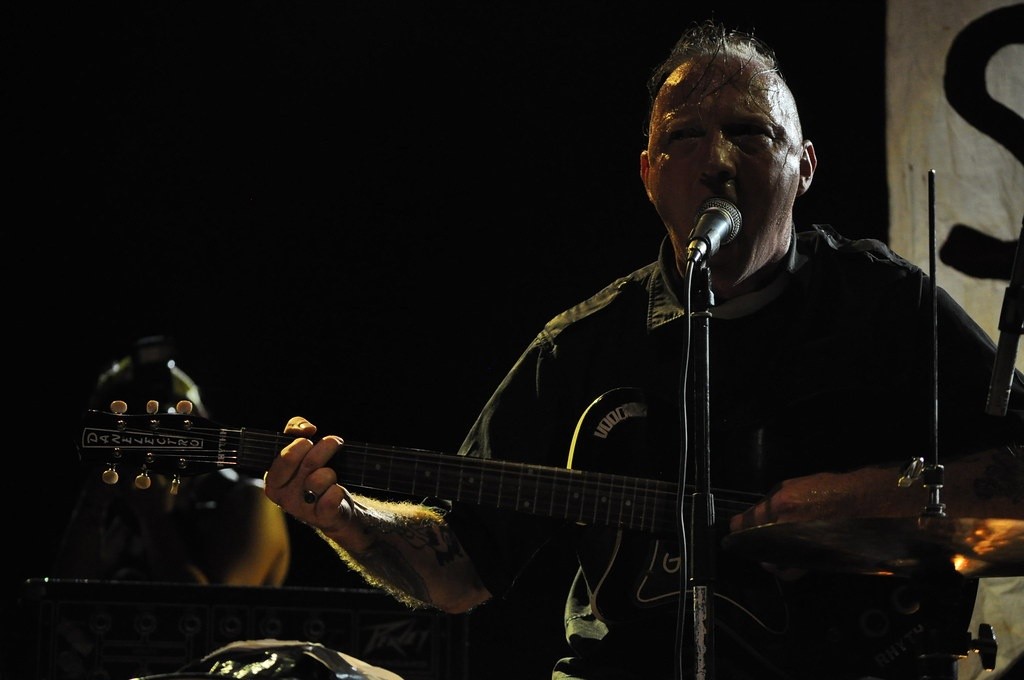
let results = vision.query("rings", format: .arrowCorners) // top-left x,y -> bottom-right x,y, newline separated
302,488 -> 319,504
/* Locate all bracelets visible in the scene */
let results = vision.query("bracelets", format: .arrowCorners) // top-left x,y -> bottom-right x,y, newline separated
321,499 -> 351,533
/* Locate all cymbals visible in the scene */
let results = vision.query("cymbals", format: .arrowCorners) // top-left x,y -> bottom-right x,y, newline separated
719,513 -> 1024,579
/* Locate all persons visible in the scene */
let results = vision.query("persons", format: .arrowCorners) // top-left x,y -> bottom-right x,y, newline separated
59,351 -> 291,588
263,24 -> 1024,680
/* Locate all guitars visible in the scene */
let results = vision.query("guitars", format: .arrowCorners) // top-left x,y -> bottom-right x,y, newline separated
69,393 -> 988,680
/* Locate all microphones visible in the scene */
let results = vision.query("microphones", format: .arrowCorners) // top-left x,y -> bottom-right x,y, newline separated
685,195 -> 745,262
